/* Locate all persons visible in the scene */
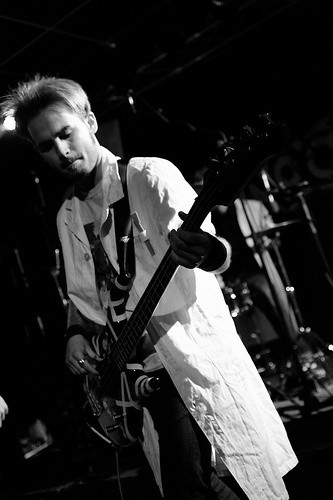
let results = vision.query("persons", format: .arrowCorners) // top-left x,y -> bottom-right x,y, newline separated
8,77 -> 300,500
193,168 -> 300,347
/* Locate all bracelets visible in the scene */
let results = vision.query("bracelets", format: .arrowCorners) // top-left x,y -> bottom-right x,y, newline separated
66,324 -> 83,340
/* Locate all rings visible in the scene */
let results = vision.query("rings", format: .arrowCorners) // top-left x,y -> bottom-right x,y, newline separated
78,358 -> 85,364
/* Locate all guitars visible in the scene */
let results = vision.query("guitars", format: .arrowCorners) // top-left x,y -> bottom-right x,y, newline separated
81,113 -> 274,451
